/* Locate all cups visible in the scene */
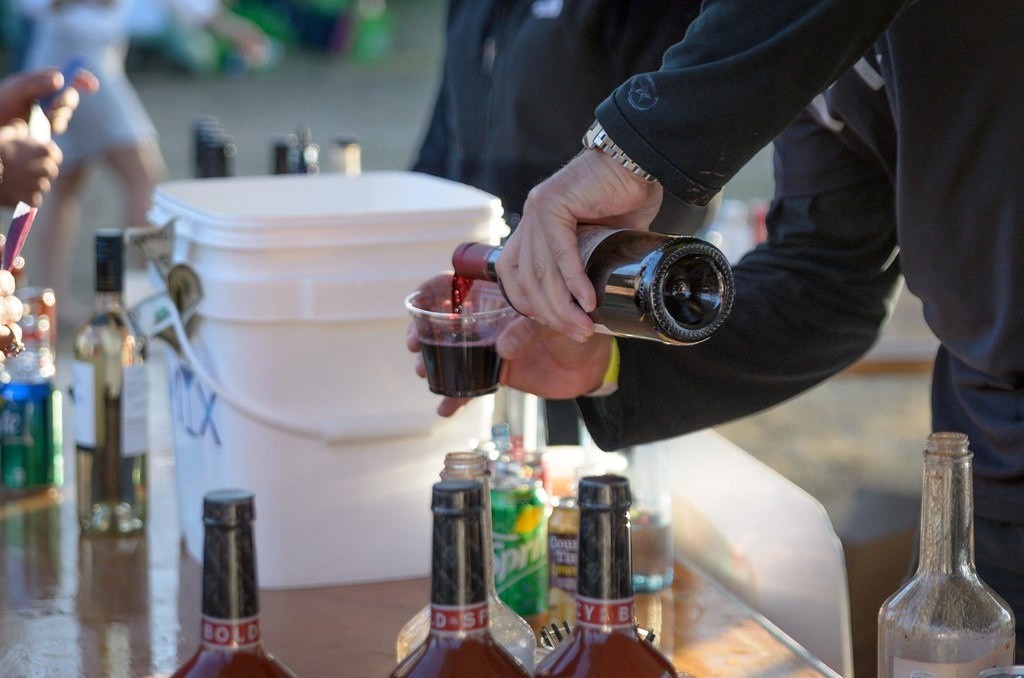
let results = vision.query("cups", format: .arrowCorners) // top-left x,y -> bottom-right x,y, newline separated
534,625 -> 660,660
629,504 -> 675,596
404,286 -> 518,398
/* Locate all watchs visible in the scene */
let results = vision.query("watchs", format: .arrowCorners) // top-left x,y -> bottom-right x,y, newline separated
582,118 -> 660,184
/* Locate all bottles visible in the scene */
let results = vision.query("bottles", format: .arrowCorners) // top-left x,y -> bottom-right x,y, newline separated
191,113 -> 360,175
390,479 -> 528,678
166,487 -> 294,678
70,229 -> 151,534
451,225 -> 735,346
396,451 -> 537,677
534,474 -> 682,678
979,665 -> 1024,678
877,431 -> 1017,678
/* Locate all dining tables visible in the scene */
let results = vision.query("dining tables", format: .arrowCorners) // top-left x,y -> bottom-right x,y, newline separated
0,486 -> 843,678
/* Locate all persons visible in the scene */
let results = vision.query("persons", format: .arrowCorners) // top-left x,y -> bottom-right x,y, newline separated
407,0 -> 723,237
406,1 -> 1024,665
0,233 -> 23,363
23,0 -> 271,334
0,67 -> 100,208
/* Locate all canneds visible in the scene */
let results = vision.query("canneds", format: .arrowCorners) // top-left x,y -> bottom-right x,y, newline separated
487,474 -> 576,616
1,366 -> 63,493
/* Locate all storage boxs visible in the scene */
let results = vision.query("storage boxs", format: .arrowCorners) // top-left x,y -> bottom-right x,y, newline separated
125,167 -> 514,590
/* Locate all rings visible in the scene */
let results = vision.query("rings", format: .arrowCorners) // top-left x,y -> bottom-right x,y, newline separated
0,326 -> 27,360
